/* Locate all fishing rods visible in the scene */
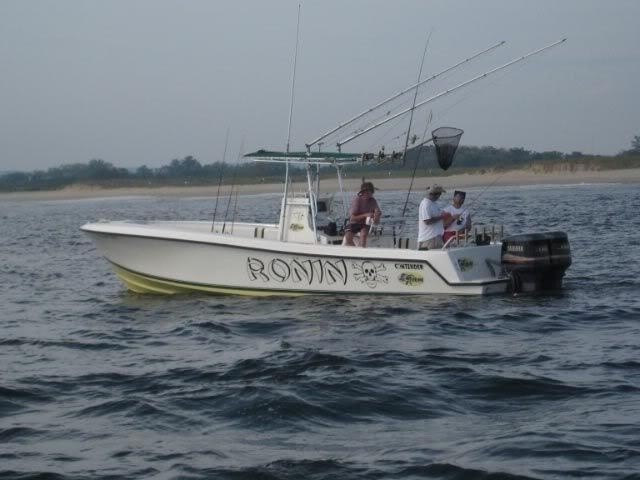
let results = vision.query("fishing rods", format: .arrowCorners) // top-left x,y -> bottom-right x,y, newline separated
445,176 -> 499,230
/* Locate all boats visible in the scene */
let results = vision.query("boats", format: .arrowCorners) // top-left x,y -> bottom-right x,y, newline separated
75,4 -> 572,297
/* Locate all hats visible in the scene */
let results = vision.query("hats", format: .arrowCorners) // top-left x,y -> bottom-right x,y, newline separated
357,182 -> 379,195
426,183 -> 447,195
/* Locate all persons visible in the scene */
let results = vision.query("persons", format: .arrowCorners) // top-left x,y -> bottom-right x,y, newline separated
342,181 -> 382,249
417,182 -> 451,249
442,189 -> 472,247
